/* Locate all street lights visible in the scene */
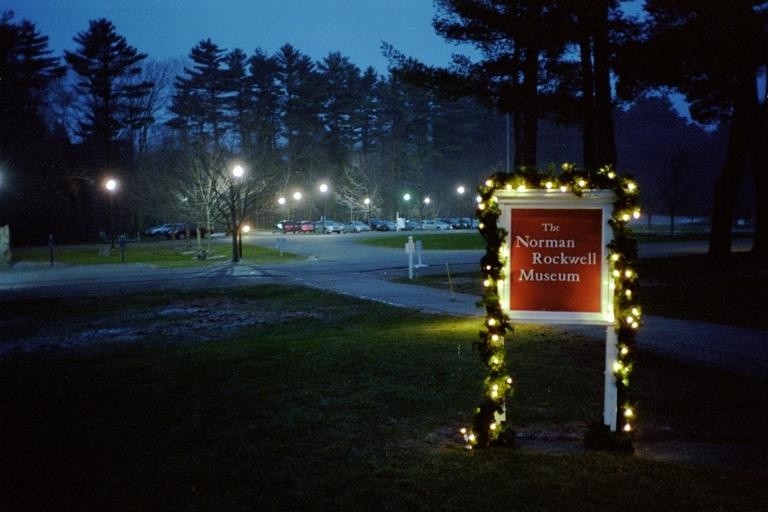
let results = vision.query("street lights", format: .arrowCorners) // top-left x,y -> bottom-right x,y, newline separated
102,178 -> 119,249
320,184 -> 327,234
456,186 -> 465,229
363,198 -> 372,231
233,165 -> 244,258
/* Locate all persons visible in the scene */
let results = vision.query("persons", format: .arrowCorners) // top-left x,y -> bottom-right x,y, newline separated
398,213 -> 407,231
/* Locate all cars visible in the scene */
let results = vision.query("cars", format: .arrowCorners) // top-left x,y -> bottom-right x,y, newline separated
145,223 -> 226,240
272,217 -> 479,234
